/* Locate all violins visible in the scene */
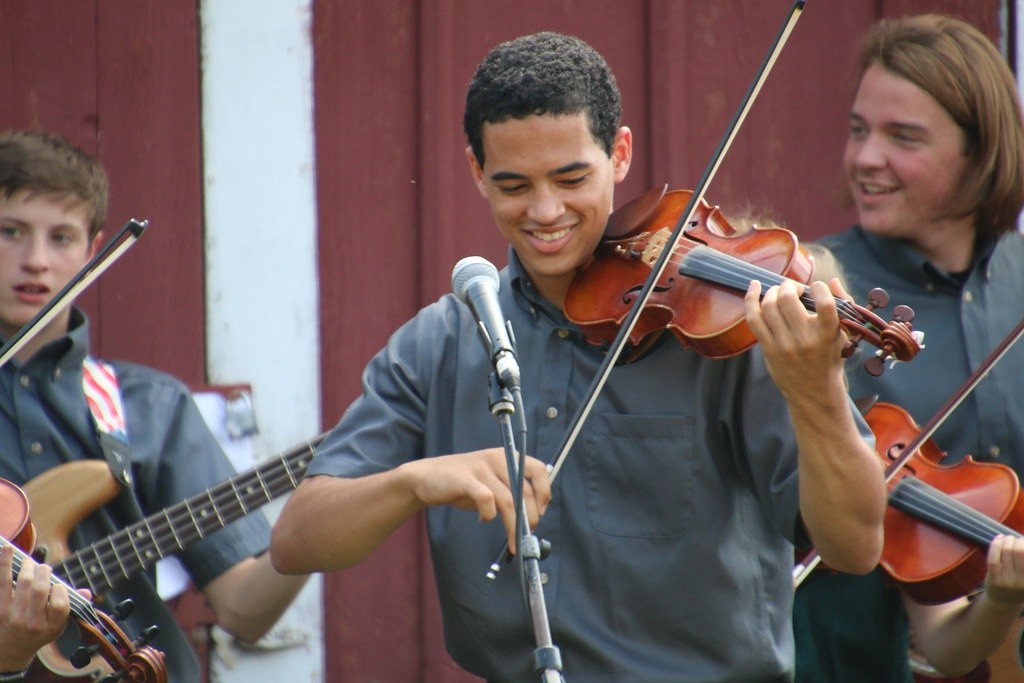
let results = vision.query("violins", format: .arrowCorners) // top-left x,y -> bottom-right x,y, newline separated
860,402 -> 1024,605
560,181 -> 927,377
0,478 -> 168,681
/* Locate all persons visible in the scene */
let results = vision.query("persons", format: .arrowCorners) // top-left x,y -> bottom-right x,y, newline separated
0,132 -> 312,683
271,32 -> 885,683
792,519 -> 1024,683
793,13 -> 1024,482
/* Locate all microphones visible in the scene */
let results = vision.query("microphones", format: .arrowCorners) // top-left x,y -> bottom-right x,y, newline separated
451,256 -> 521,396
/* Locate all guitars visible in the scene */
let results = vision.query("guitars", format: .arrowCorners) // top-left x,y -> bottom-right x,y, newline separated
23,426 -> 332,683
